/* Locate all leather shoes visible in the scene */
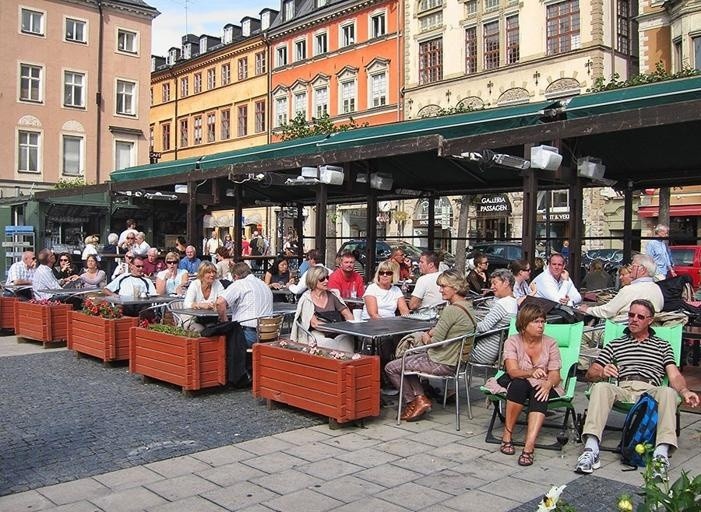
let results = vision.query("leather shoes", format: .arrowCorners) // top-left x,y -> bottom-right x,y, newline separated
396,395 -> 432,422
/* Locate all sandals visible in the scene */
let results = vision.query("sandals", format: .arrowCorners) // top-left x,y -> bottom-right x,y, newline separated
519,449 -> 534,466
500,438 -> 515,455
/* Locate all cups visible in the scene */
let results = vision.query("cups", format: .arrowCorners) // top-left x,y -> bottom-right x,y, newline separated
133,285 -> 140,298
352,308 -> 362,322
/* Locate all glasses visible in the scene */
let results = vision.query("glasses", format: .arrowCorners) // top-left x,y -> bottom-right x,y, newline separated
628,312 -> 652,320
379,270 -> 393,276
318,275 -> 329,282
523,268 -> 531,273
481,262 -> 488,265
31,237 -> 179,269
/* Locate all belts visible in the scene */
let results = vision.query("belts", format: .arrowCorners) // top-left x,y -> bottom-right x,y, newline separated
618,373 -> 657,387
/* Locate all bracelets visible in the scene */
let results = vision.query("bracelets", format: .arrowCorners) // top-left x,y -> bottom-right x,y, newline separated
548,381 -> 555,388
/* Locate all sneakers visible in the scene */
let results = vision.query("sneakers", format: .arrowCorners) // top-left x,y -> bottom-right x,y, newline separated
446,390 -> 456,399
652,454 -> 670,482
574,448 -> 600,474
382,389 -> 400,396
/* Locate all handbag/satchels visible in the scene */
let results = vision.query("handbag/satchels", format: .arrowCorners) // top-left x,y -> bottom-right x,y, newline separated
315,311 -> 346,339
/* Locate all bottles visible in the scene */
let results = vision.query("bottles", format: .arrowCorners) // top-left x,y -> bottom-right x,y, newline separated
609,358 -> 620,386
350,281 -> 357,299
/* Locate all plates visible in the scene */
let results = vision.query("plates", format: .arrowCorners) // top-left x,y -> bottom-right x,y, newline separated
147,294 -> 160,297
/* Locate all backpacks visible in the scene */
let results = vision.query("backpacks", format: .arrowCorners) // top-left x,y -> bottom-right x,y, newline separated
618,392 -> 658,468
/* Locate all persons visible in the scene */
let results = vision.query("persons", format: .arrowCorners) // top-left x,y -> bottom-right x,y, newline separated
7,218 -> 677,352
574,299 -> 701,482
473,268 -> 520,365
500,306 -> 566,465
217,261 -> 274,388
384,269 -> 477,422
